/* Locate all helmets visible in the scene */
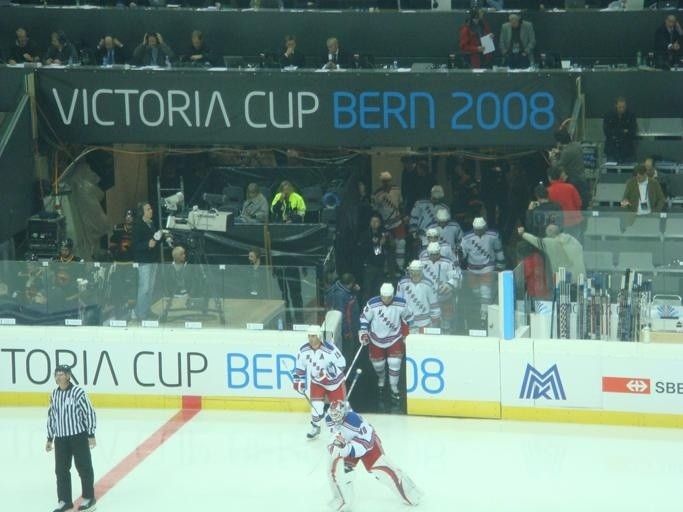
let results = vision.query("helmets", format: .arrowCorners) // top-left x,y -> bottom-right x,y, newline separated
327,400 -> 347,422
306,329 -> 322,338
426,228 -> 439,241
380,172 -> 392,181
436,209 -> 450,221
472,217 -> 486,230
427,242 -> 440,256
55,365 -> 72,378
406,260 -> 424,277
430,185 -> 444,199
380,282 -> 394,299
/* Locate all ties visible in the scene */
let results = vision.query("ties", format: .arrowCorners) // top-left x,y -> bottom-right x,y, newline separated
332,54 -> 337,63
109,50 -> 113,65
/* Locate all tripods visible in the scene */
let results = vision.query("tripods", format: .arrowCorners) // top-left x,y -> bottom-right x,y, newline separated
159,236 -> 226,325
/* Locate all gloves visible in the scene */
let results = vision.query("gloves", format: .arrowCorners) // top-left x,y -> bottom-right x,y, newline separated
438,280 -> 456,296
359,330 -> 369,346
334,432 -> 352,457
402,325 -> 409,336
293,375 -> 305,396
411,231 -> 417,239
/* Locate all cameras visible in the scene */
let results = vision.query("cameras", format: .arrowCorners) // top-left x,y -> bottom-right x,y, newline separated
164,192 -> 234,232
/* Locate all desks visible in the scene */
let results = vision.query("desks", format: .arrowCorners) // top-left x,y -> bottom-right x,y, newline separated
1,275 -> 100,326
122,298 -> 287,331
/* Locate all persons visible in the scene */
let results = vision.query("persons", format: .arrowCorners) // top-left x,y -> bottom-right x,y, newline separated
93,32 -> 131,66
318,37 -> 356,69
293,325 -> 353,441
422,210 -> 461,250
549,130 -> 585,182
46,365 -> 96,512
621,166 -> 664,229
270,181 -> 307,223
48,239 -> 85,290
101,208 -> 135,263
654,15 -> 683,65
409,186 -> 450,240
372,171 -> 404,279
516,240 -> 549,309
42,31 -> 78,65
545,165 -> 585,227
404,160 -> 437,212
276,33 -> 304,69
499,15 -> 537,69
164,247 -> 194,295
358,283 -> 414,403
458,8 -> 497,68
360,212 -> 395,295
426,229 -> 463,283
418,243 -> 461,332
325,400 -> 420,512
602,97 -> 636,173
234,184 -> 269,224
132,31 -> 175,67
517,226 -> 588,289
325,273 -> 360,342
645,157 -> 667,195
183,29 -> 219,67
459,217 -> 504,329
2,27 -> 41,64
396,260 -> 440,333
525,185 -> 565,235
243,248 -> 266,298
131,200 -> 159,322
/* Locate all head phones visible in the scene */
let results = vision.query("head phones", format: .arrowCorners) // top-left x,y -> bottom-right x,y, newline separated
138,201 -> 148,217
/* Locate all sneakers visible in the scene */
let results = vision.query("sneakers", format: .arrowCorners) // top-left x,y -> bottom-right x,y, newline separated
54,500 -> 73,512
390,386 -> 400,398
378,376 -> 386,389
307,426 -> 320,438
79,496 -> 96,512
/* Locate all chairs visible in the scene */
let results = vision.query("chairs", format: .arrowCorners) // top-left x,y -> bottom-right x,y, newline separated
596,174 -> 629,207
582,250 -> 614,271
663,217 -> 682,239
623,217 -> 662,245
615,252 -> 654,271
584,217 -> 620,241
